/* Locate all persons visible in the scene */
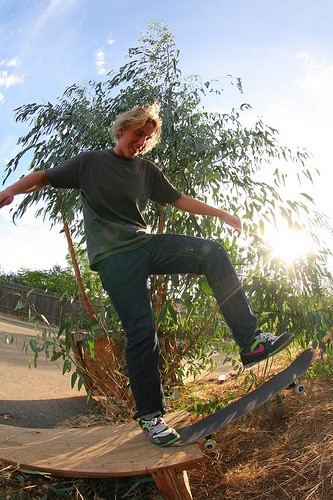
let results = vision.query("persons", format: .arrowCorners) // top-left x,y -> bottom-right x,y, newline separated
1,103 -> 297,451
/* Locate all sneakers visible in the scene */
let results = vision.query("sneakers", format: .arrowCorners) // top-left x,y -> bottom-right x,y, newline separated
139,414 -> 180,447
239,330 -> 295,368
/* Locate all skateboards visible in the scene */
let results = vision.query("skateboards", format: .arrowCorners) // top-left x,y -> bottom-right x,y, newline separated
167,348 -> 312,450
